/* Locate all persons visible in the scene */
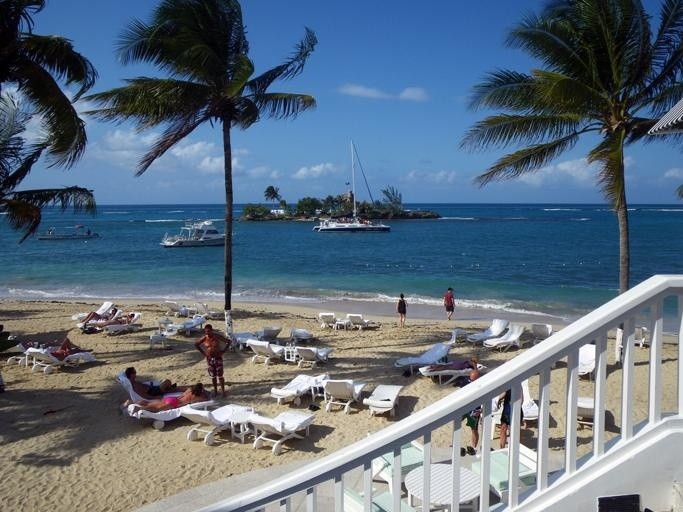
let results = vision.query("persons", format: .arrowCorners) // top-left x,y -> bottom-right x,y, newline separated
447,369 -> 483,455
442,287 -> 456,320
80,308 -> 118,324
193,323 -> 233,400
395,293 -> 408,327
49,338 -> 93,361
120,383 -> 212,418
426,356 -> 478,372
497,385 -> 524,450
95,313 -> 135,328
19,338 -> 62,350
124,366 -> 204,400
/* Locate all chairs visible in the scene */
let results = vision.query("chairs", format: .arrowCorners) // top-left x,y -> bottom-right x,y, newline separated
270,375 -> 404,418
250,409 -> 315,455
72,302 -> 142,336
115,370 -> 216,430
6,342 -> 95,373
231,327 -> 334,370
335,422 -> 545,512
179,403 -> 255,445
158,300 -> 224,336
394,317 -> 596,438
319,313 -> 375,332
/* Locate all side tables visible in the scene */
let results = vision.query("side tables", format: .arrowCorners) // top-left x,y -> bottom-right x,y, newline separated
231,411 -> 259,444
149,333 -> 166,351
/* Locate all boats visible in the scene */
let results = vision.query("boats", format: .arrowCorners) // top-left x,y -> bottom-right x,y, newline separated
37,224 -> 99,240
312,139 -> 390,232
159,221 -> 225,247
